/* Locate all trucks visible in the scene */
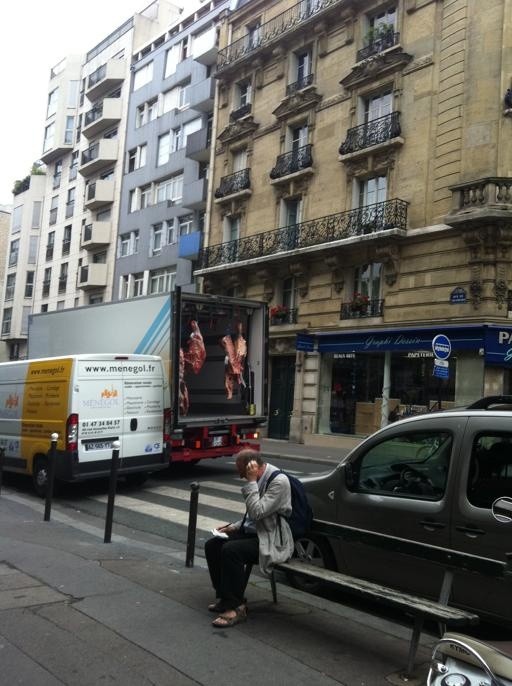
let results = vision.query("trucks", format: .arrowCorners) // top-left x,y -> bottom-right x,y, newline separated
28,285 -> 269,474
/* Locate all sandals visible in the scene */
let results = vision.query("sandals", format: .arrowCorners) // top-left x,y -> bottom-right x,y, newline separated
212,606 -> 250,629
208,596 -> 248,615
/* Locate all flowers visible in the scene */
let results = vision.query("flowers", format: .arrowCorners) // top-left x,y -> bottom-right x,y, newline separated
270,304 -> 288,315
349,291 -> 370,317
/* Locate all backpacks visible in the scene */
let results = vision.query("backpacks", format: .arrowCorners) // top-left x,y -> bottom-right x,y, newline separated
263,469 -> 313,540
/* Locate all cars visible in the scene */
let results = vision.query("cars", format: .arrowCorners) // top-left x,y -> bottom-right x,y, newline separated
296,395 -> 512,627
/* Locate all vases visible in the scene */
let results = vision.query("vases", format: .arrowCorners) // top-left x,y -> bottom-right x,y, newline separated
351,303 -> 363,311
274,314 -> 286,318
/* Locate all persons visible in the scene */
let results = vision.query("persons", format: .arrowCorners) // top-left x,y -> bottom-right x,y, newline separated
203,447 -> 297,629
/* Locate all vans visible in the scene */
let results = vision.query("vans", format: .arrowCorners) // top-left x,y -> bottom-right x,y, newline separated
0,353 -> 171,499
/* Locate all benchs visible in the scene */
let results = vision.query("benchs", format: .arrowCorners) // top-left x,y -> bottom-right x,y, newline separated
241,557 -> 478,681
